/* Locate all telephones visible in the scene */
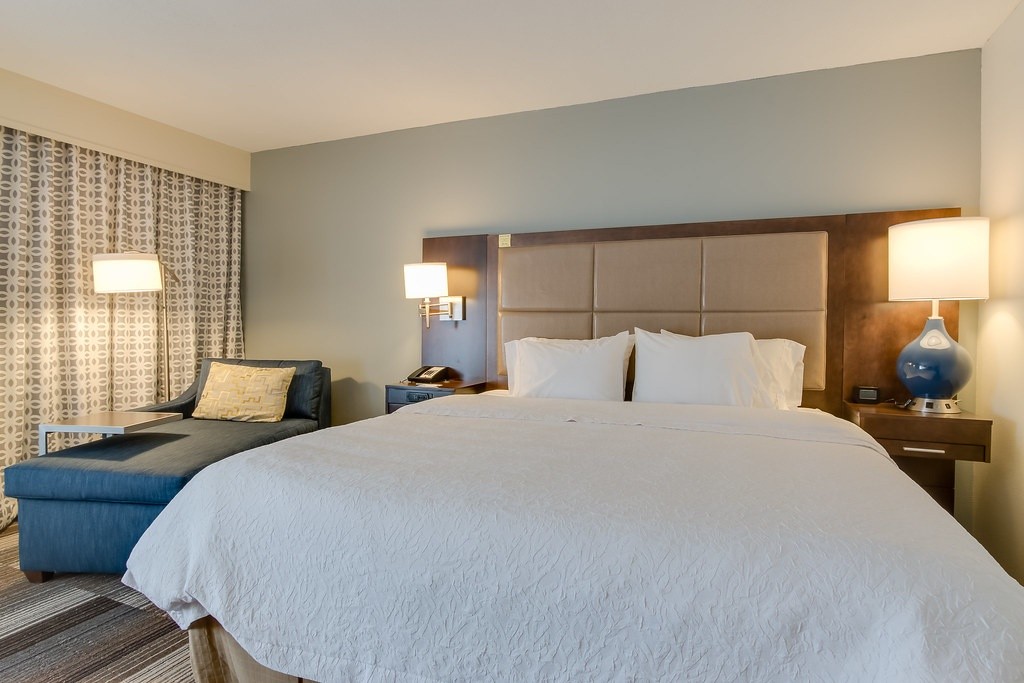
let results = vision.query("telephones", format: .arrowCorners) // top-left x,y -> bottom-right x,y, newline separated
408,366 -> 449,383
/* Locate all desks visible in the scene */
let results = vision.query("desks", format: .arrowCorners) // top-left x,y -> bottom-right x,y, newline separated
40,410 -> 183,455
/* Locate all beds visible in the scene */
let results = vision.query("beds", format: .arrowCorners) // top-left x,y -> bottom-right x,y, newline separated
121,206 -> 1024,683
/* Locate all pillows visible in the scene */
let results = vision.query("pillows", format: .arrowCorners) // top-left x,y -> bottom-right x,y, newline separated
504,333 -> 635,403
634,327 -> 785,411
661,328 -> 807,410
192,362 -> 296,423
513,330 -> 629,402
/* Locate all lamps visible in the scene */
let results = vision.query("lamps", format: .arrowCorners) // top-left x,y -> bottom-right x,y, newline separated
92,251 -> 180,401
887,215 -> 991,414
404,262 -> 466,329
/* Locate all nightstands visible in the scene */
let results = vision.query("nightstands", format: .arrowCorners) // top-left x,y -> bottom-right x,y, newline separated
843,400 -> 993,463
385,381 -> 484,414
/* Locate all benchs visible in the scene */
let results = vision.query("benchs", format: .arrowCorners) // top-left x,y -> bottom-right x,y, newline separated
4,357 -> 331,582
